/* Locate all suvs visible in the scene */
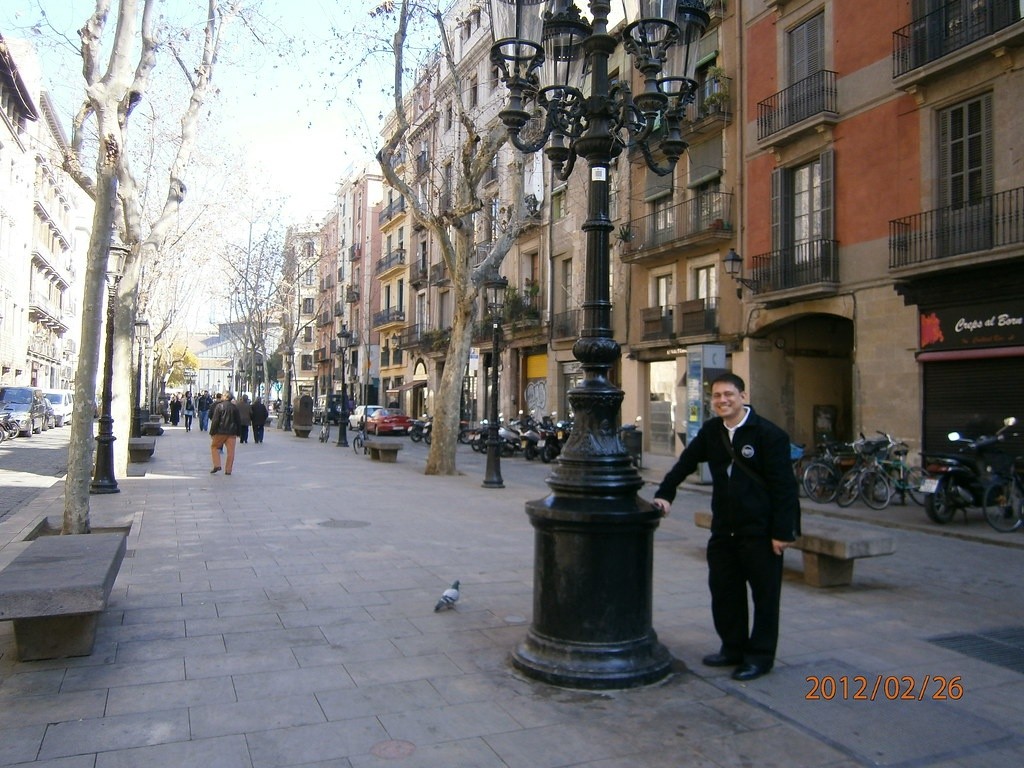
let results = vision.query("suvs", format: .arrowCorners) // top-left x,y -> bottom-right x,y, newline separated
312,395 -> 342,427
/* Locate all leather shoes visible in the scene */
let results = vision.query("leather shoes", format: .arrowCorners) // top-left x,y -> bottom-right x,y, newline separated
702,654 -> 752,668
731,660 -> 772,681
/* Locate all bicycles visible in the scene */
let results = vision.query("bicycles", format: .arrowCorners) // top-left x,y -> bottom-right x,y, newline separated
353,421 -> 371,455
792,430 -> 928,509
984,457 -> 1024,533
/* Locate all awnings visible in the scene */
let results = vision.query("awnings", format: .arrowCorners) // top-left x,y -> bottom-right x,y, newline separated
386,380 -> 427,393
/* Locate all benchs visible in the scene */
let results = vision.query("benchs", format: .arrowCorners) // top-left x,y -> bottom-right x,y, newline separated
143,415 -> 162,436
0,531 -> 127,662
363,440 -> 404,463
129,438 -> 156,463
693,508 -> 898,587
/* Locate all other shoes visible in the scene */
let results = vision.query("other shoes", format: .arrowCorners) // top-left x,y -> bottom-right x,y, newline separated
210,466 -> 221,473
225,473 -> 231,475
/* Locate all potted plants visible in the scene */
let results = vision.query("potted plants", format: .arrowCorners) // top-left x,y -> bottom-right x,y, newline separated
613,224 -> 637,252
704,66 -> 730,113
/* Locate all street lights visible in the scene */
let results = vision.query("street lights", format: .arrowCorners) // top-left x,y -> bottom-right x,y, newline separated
482,273 -> 508,489
88,224 -> 131,494
283,346 -> 295,431
336,324 -> 348,447
132,318 -> 150,438
487,0 -> 713,687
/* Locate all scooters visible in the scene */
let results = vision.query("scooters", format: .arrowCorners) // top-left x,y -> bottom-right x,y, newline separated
916,415 -> 1024,524
406,413 -> 432,445
456,410 -> 574,464
620,416 -> 642,467
0,412 -> 21,443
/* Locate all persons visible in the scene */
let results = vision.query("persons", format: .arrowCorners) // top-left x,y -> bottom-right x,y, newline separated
209,390 -> 241,475
652,373 -> 802,681
157,389 -> 268,443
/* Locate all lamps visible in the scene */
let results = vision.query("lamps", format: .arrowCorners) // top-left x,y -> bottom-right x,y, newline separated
723,248 -> 761,290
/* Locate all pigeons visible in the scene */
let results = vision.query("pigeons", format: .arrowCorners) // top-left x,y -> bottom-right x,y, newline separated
433,580 -> 462,611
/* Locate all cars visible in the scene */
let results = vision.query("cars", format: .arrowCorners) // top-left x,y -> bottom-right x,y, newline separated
367,408 -> 413,436
348,405 -> 384,431
0,387 -> 49,437
44,398 -> 56,429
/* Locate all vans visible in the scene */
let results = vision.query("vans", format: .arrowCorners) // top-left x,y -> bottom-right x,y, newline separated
44,389 -> 75,427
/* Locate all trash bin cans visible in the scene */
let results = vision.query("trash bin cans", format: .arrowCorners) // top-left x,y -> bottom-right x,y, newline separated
293,395 -> 313,437
